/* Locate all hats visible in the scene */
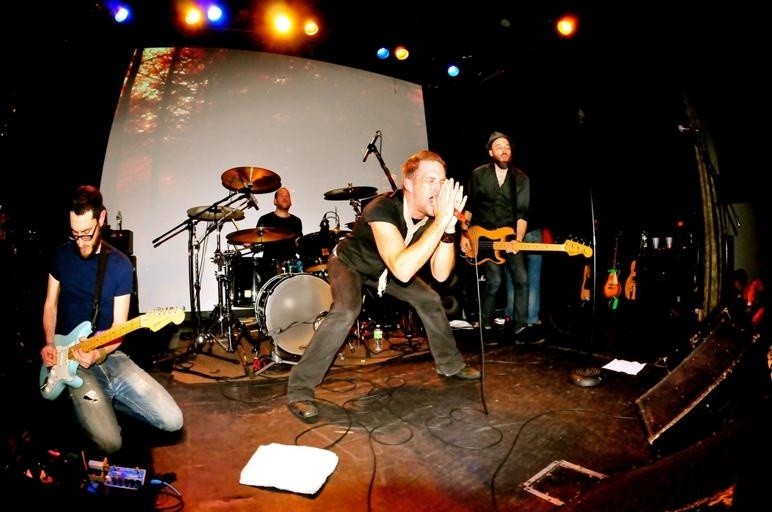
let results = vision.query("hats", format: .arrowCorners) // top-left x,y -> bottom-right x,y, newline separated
485,131 -> 512,149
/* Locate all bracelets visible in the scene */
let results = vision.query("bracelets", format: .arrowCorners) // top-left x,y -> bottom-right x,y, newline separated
441,232 -> 456,244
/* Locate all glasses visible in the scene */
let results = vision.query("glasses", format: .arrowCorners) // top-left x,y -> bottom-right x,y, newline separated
68,223 -> 99,242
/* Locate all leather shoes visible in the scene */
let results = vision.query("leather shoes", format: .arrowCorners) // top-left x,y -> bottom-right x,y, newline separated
437,359 -> 481,381
289,392 -> 320,424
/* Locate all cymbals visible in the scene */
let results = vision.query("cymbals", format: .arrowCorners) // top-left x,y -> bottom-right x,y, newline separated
221,167 -> 282,194
324,181 -> 378,201
187,206 -> 245,222
225,227 -> 298,245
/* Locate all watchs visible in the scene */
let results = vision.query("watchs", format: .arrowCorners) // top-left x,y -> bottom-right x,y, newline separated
94,347 -> 106,366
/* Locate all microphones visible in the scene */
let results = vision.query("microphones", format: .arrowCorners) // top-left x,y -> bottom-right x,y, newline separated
453,209 -> 471,228
676,123 -> 702,136
362,132 -> 382,164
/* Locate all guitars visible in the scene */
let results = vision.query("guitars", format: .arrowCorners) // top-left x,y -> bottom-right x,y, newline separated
604,230 -> 623,301
580,235 -> 596,305
459,224 -> 594,267
38,305 -> 186,400
624,230 -> 648,303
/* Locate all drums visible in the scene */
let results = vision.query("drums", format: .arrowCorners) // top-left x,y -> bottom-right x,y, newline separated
234,256 -> 277,290
295,229 -> 352,272
254,273 -> 333,356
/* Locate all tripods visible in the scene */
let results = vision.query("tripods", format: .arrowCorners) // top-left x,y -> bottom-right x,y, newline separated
193,200 -> 235,353
153,195 -> 223,354
193,249 -> 260,353
152,191 -> 239,368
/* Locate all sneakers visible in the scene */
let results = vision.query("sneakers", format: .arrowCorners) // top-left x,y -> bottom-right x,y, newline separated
514,326 -> 546,345
482,328 -> 500,346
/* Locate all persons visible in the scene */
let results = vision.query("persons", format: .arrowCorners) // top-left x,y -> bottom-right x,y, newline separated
730,271 -> 772,347
288,151 -> 482,421
40,185 -> 184,456
258,187 -> 301,256
462,131 -> 531,349
